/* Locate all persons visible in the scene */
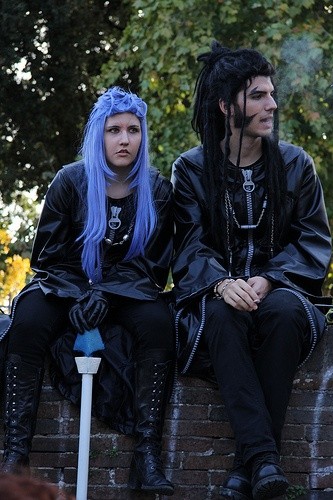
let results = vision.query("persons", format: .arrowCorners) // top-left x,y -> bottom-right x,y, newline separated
166,42 -> 333,492
0,86 -> 186,497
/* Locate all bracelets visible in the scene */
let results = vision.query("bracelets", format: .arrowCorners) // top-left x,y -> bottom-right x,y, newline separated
220,280 -> 234,296
214,279 -> 224,297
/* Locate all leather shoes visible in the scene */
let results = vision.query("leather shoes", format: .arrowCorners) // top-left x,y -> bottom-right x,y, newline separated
217,451 -> 290,500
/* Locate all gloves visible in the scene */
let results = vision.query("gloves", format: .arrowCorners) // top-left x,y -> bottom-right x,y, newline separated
68,290 -> 110,335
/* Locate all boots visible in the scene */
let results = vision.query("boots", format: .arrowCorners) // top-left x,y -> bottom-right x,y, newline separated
126,358 -> 175,496
0,353 -> 46,481
